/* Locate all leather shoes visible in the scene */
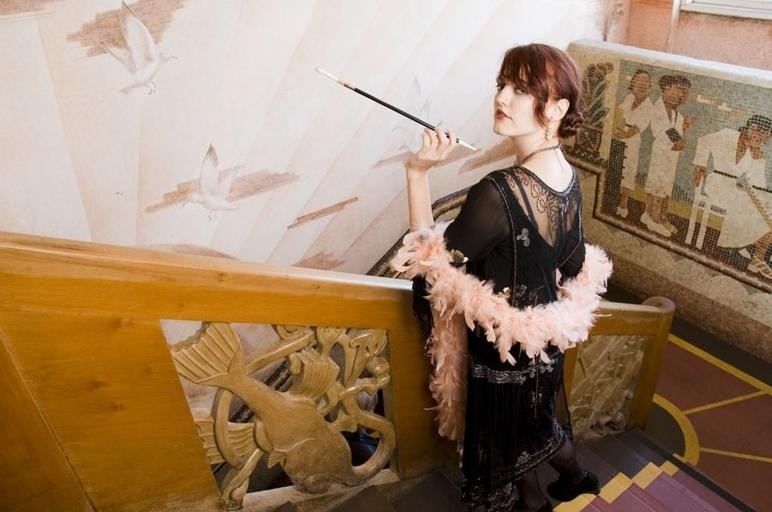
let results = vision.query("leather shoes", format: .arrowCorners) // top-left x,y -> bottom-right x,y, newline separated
545,468 -> 603,502
513,497 -> 555,512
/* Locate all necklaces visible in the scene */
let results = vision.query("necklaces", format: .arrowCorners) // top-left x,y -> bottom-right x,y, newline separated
520,145 -> 561,164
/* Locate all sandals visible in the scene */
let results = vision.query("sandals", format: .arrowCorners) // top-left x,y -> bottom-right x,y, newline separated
739,248 -> 771,281
616,204 -> 678,239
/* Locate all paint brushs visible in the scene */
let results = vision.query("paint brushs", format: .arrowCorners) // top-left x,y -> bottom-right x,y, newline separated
314,67 -> 479,152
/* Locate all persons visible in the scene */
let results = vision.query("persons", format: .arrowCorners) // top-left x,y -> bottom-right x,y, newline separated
405,43 -> 600,512
614,70 -> 771,278
